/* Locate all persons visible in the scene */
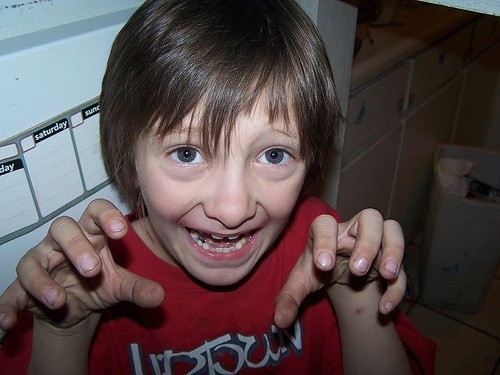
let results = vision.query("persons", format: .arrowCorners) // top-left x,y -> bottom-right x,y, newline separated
0,1 -> 438,375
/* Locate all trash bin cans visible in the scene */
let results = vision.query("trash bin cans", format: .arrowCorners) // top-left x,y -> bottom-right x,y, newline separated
411,143 -> 500,322
